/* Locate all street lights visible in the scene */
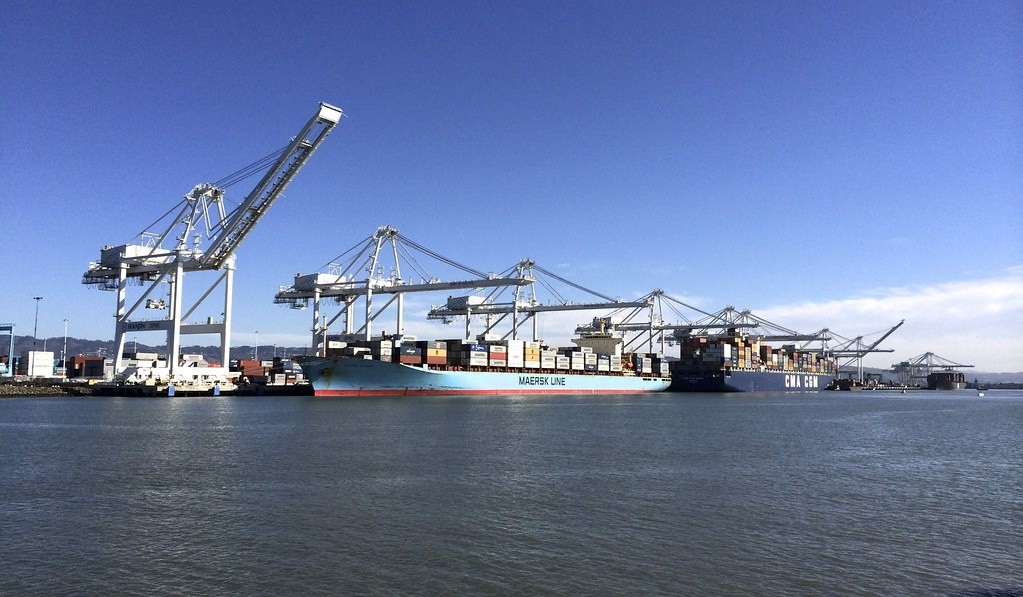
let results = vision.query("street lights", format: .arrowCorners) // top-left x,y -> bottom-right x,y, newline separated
31,296 -> 43,378
254,331 -> 259,361
63,318 -> 69,381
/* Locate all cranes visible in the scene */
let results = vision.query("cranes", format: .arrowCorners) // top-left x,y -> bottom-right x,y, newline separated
83,103 -> 345,385
892,352 -> 975,388
657,306 -> 910,383
273,223 -> 535,357
425,257 -> 655,343
572,288 -> 760,350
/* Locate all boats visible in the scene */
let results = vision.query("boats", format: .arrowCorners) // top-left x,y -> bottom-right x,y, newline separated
668,337 -> 837,394
290,317 -> 674,399
